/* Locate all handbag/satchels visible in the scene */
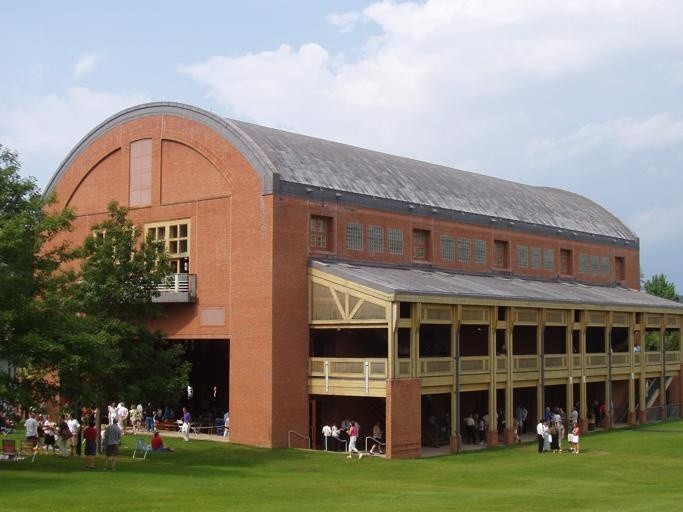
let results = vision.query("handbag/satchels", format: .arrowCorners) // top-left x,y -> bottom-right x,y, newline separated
548,435 -> 552,443
62,427 -> 73,439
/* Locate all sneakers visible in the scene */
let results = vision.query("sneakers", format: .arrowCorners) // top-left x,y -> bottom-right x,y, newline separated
347,454 -> 363,460
369,450 -> 384,455
556,448 -> 579,453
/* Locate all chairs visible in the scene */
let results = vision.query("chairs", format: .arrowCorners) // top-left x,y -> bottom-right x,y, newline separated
0,438 -> 39,465
132,439 -> 152,463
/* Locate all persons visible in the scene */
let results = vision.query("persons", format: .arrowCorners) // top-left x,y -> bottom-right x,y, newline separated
636,344 -> 640,352
320,396 -> 616,460
496,343 -> 507,357
0,398 -> 230,472
633,344 -> 638,352
649,342 -> 657,350
